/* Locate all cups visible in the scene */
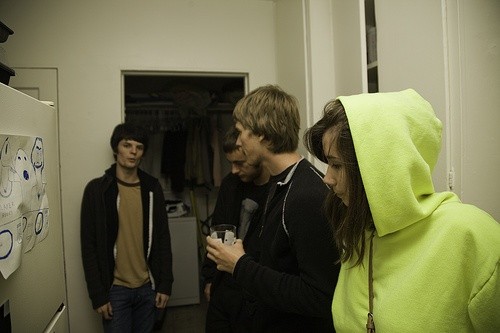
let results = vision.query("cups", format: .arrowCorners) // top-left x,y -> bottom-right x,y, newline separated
210,225 -> 236,248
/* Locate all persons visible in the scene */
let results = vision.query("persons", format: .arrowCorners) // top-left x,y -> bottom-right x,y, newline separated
79,124 -> 173,333
202,86 -> 348,333
303,88 -> 500,333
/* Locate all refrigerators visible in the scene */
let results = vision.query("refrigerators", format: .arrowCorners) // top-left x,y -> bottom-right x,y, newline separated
0,81 -> 69,333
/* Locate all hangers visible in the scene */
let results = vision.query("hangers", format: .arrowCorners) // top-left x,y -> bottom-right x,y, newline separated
126,109 -> 202,135
